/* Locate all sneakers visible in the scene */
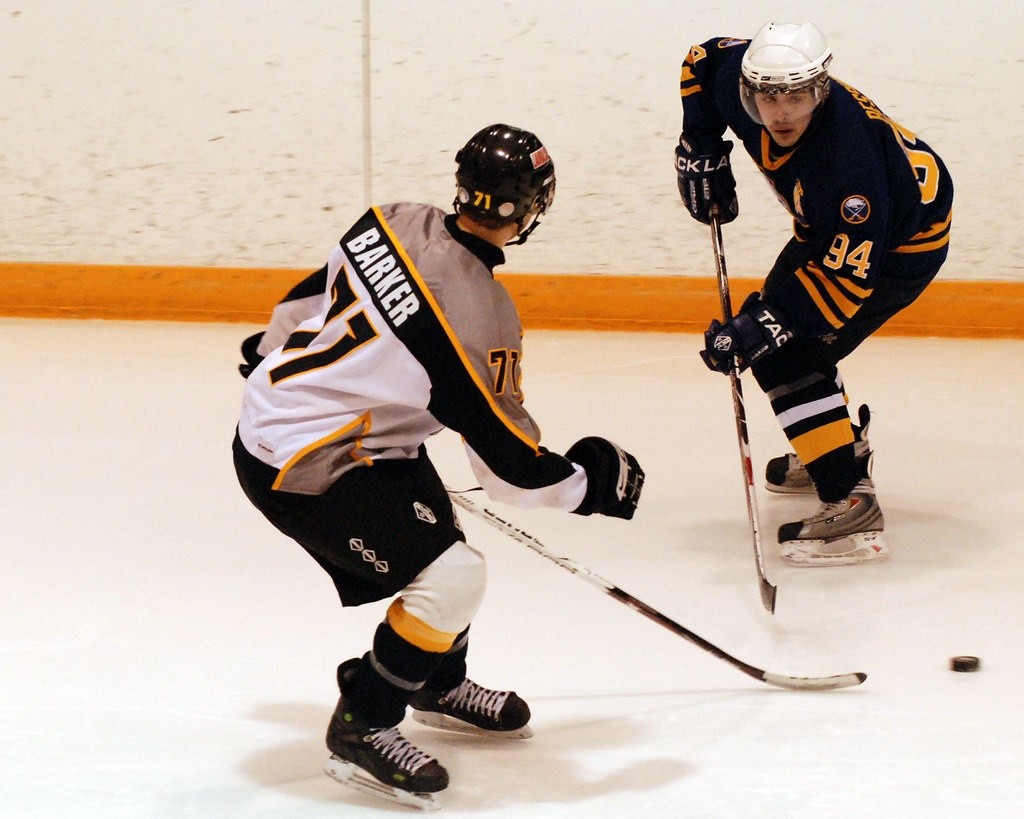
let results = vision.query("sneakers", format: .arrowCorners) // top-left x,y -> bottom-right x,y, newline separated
324,694 -> 451,809
763,404 -> 875,497
408,666 -> 535,741
776,475 -> 891,567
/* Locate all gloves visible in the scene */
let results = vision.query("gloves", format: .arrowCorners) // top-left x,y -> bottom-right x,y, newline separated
240,333 -> 263,377
567,436 -> 647,522
673,134 -> 740,226
698,292 -> 794,376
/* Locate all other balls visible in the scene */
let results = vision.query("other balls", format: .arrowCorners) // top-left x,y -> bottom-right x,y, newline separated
949,655 -> 979,673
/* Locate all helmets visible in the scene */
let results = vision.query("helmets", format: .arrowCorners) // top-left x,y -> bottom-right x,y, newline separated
738,16 -> 835,88
455,123 -> 558,212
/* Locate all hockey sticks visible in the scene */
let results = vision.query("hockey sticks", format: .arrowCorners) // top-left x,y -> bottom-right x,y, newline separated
709,209 -> 781,614
447,490 -> 867,690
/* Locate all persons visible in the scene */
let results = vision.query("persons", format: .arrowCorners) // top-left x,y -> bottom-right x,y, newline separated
231,125 -> 645,812
673,20 -> 955,566
